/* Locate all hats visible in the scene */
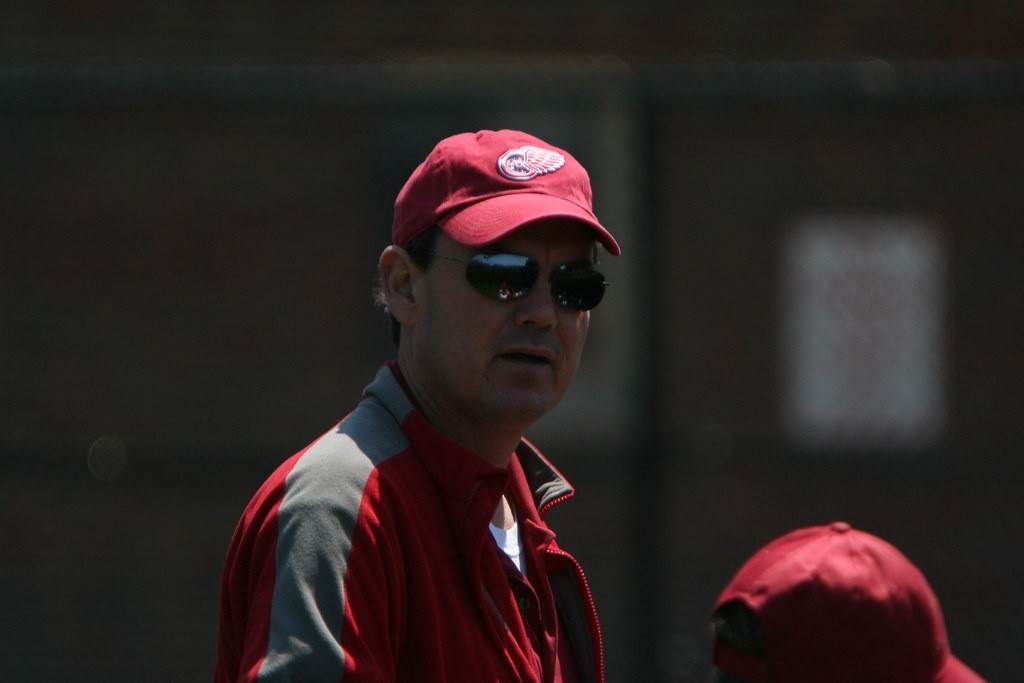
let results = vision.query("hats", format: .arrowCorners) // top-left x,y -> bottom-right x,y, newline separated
392,130 -> 621,256
711,520 -> 986,683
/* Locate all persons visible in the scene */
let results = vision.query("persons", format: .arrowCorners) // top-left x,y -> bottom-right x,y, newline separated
711,523 -> 986,683
214,130 -> 621,683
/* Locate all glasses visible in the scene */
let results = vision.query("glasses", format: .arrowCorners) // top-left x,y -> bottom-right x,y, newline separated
418,250 -> 611,312
